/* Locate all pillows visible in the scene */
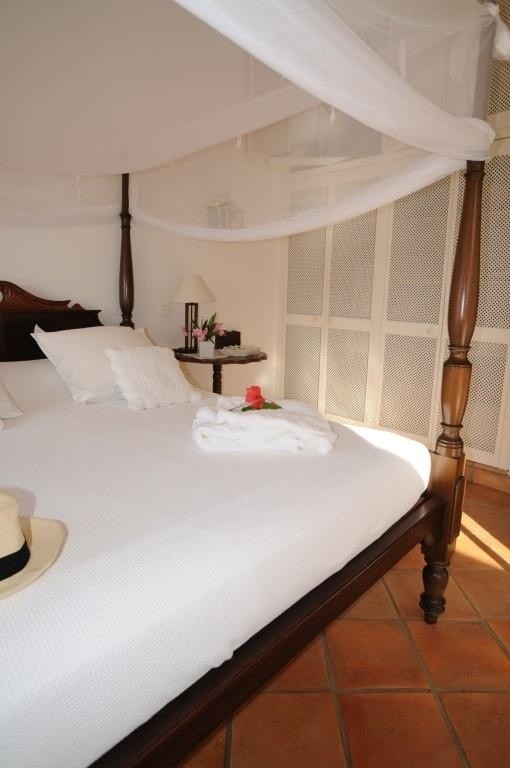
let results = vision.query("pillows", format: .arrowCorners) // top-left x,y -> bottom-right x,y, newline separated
0,375 -> 26,419
103,342 -> 203,411
29,321 -> 156,408
0,415 -> 7,429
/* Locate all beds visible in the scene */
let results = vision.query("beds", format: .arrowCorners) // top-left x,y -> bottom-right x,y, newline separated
0,1 -> 506,767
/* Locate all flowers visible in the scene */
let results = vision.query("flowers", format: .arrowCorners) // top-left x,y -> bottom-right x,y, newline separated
183,311 -> 222,340
229,385 -> 282,413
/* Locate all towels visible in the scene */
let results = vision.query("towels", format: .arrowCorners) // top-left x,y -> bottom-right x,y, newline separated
192,390 -> 341,457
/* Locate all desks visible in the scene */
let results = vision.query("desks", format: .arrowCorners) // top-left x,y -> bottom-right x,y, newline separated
171,346 -> 270,396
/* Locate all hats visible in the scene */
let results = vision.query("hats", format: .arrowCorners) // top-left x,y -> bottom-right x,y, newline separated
0,492 -> 64,602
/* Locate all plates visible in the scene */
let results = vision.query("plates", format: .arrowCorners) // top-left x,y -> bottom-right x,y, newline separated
218,344 -> 262,357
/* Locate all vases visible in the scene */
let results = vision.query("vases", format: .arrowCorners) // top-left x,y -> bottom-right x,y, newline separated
198,340 -> 216,357
